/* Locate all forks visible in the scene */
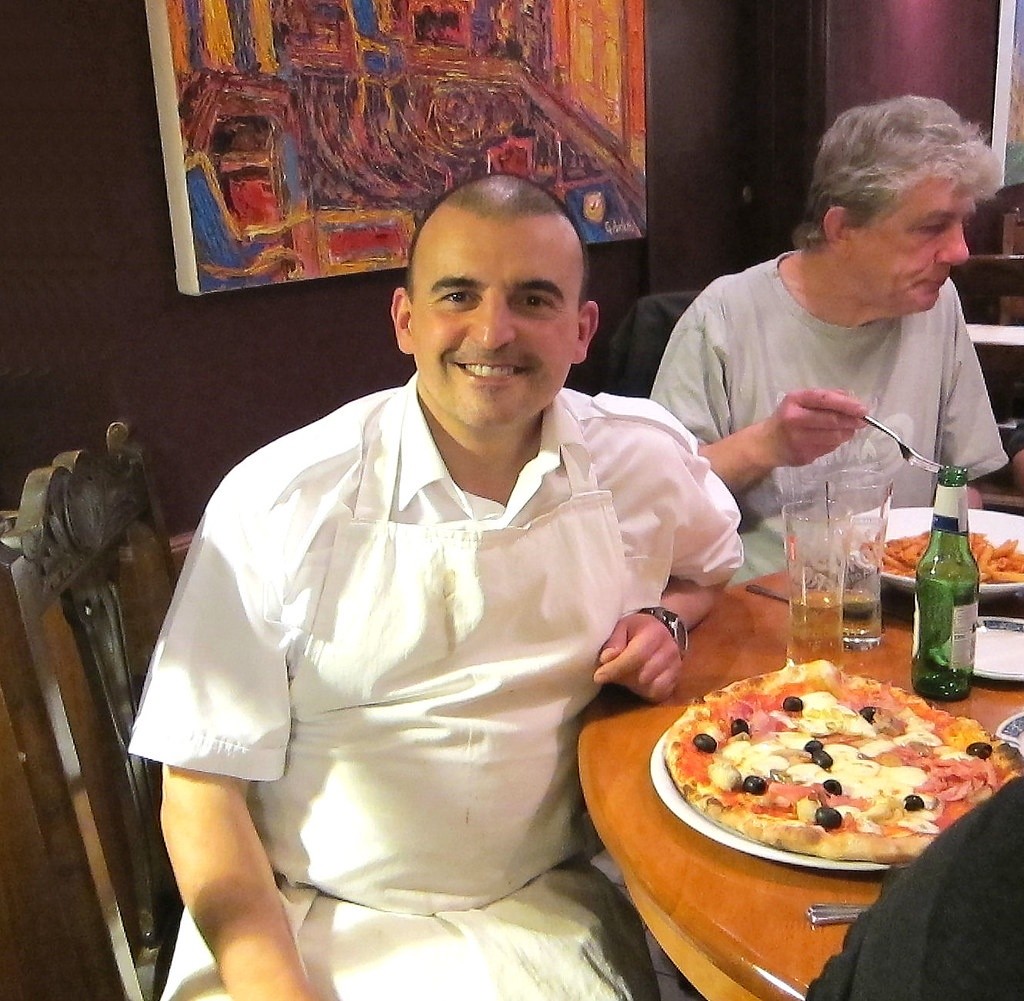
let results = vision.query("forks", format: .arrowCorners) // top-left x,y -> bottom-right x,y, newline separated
859,414 -> 950,477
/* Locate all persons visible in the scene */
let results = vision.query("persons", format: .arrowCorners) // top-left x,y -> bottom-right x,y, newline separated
127,174 -> 747,1001
648,95 -> 1011,533
803,774 -> 1024,1001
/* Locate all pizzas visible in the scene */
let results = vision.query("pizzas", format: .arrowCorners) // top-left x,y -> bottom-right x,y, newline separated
649,661 -> 1013,871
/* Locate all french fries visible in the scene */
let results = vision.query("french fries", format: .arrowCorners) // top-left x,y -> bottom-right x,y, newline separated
859,530 -> 1024,585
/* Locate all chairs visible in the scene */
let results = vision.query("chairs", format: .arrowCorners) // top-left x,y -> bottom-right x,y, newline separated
11,416 -> 184,1001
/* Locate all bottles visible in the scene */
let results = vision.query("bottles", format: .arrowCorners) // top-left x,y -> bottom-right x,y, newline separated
911,466 -> 980,702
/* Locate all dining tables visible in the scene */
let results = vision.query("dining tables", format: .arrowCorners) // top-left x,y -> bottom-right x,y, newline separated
579,567 -> 1024,1001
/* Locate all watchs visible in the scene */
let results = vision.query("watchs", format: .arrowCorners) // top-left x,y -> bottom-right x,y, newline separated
640,607 -> 689,660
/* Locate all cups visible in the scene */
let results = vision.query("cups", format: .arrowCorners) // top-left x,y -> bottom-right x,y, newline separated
781,497 -> 851,670
822,469 -> 895,651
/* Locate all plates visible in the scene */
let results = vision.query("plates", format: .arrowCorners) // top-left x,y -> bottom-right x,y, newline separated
995,711 -> 1024,759
923,616 -> 1024,685
838,504 -> 1024,601
651,675 -> 950,874
